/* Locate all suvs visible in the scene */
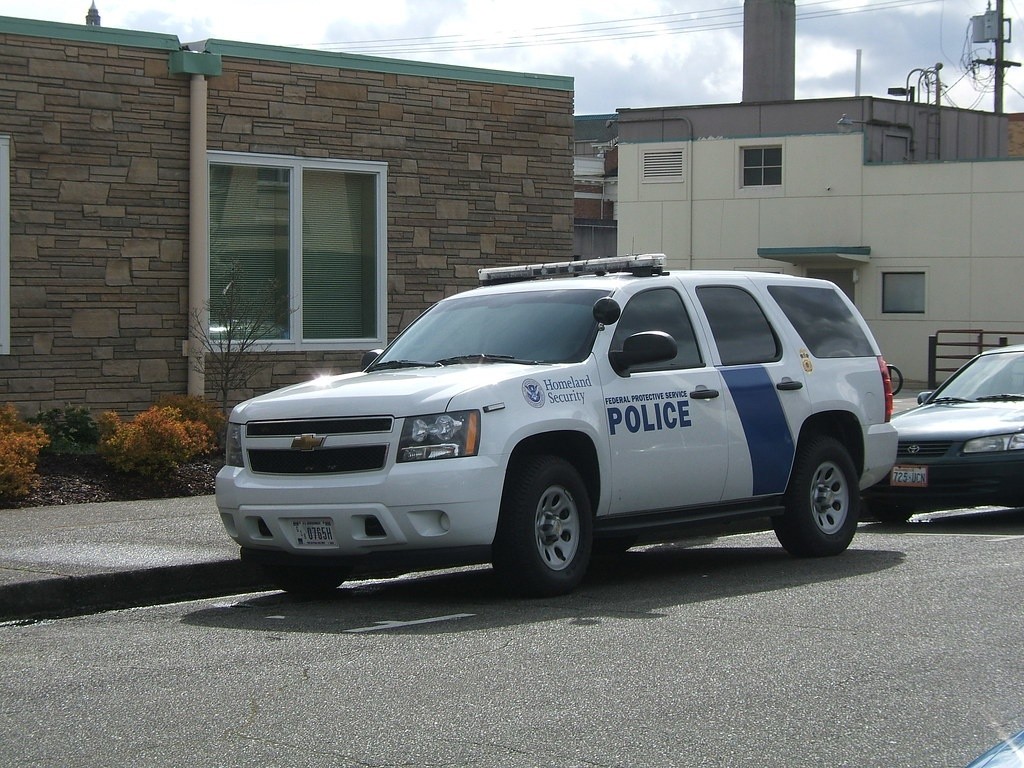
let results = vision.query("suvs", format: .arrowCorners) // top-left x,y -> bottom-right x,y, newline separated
217,254 -> 906,594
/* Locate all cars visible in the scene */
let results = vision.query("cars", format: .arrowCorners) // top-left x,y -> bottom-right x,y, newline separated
870,344 -> 1024,527
